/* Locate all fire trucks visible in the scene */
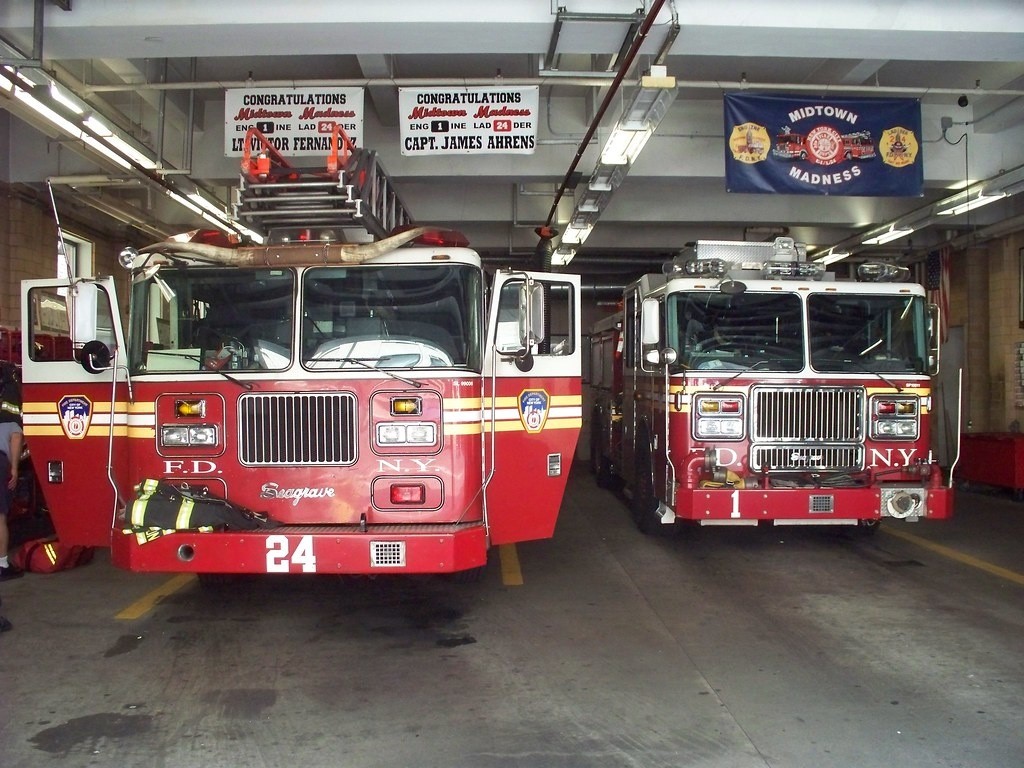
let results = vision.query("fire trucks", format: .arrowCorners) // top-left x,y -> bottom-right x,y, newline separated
839,129 -> 875,160
585,240 -> 957,531
772,134 -> 808,160
23,123 -> 583,576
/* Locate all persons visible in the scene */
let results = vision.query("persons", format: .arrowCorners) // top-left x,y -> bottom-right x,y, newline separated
0,368 -> 22,426
698,321 -> 736,353
0,422 -> 24,582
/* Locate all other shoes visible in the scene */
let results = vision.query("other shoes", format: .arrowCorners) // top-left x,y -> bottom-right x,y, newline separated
0,561 -> 24,581
0,616 -> 12,631
